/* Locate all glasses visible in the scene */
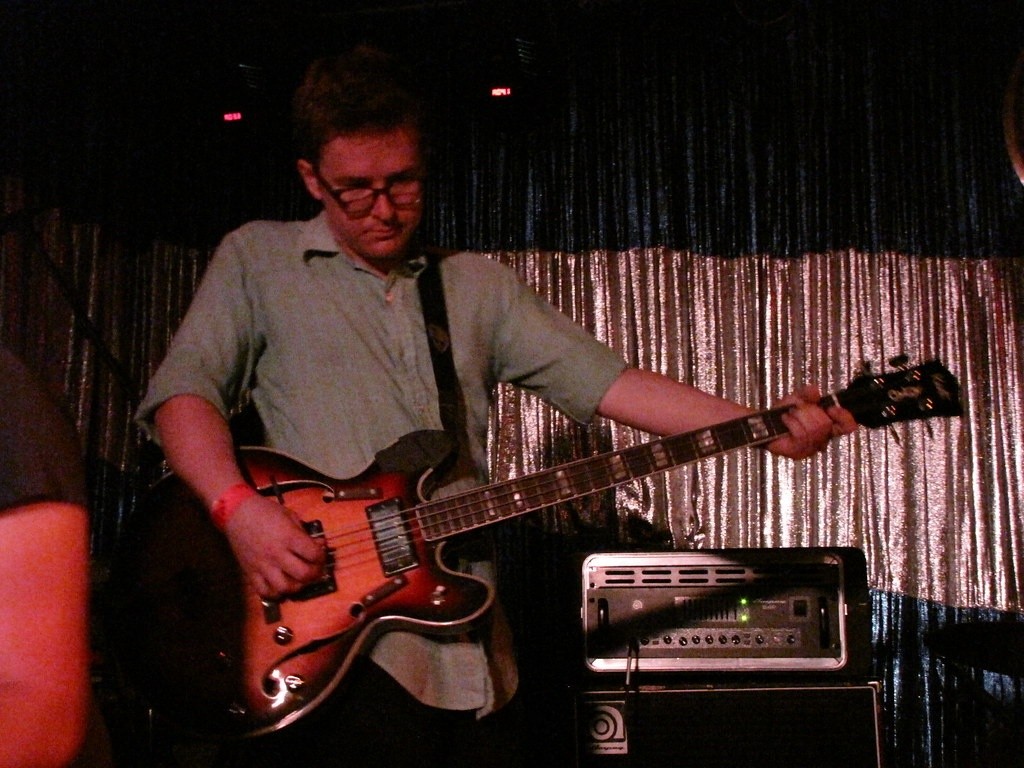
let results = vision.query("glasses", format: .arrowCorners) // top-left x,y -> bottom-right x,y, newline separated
311,162 -> 433,206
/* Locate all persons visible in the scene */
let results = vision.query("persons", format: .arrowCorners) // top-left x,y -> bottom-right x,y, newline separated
0,343 -> 112,768
137,48 -> 855,768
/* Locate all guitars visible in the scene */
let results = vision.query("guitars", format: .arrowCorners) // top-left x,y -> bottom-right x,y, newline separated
104,354 -> 964,746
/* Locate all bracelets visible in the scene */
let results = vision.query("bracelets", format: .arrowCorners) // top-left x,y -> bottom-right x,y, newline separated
211,477 -> 258,530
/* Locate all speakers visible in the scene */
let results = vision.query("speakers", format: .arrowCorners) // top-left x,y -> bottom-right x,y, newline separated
565,676 -> 883,767
